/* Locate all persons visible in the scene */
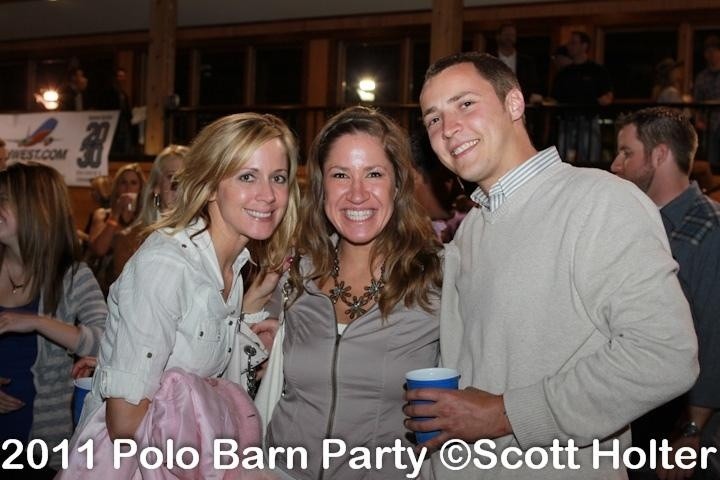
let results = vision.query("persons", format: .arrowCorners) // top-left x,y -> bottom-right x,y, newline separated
1,23 -> 720,480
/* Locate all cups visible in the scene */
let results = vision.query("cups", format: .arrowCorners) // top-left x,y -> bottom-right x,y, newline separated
123,193 -> 137,212
405,367 -> 461,444
73,377 -> 93,431
